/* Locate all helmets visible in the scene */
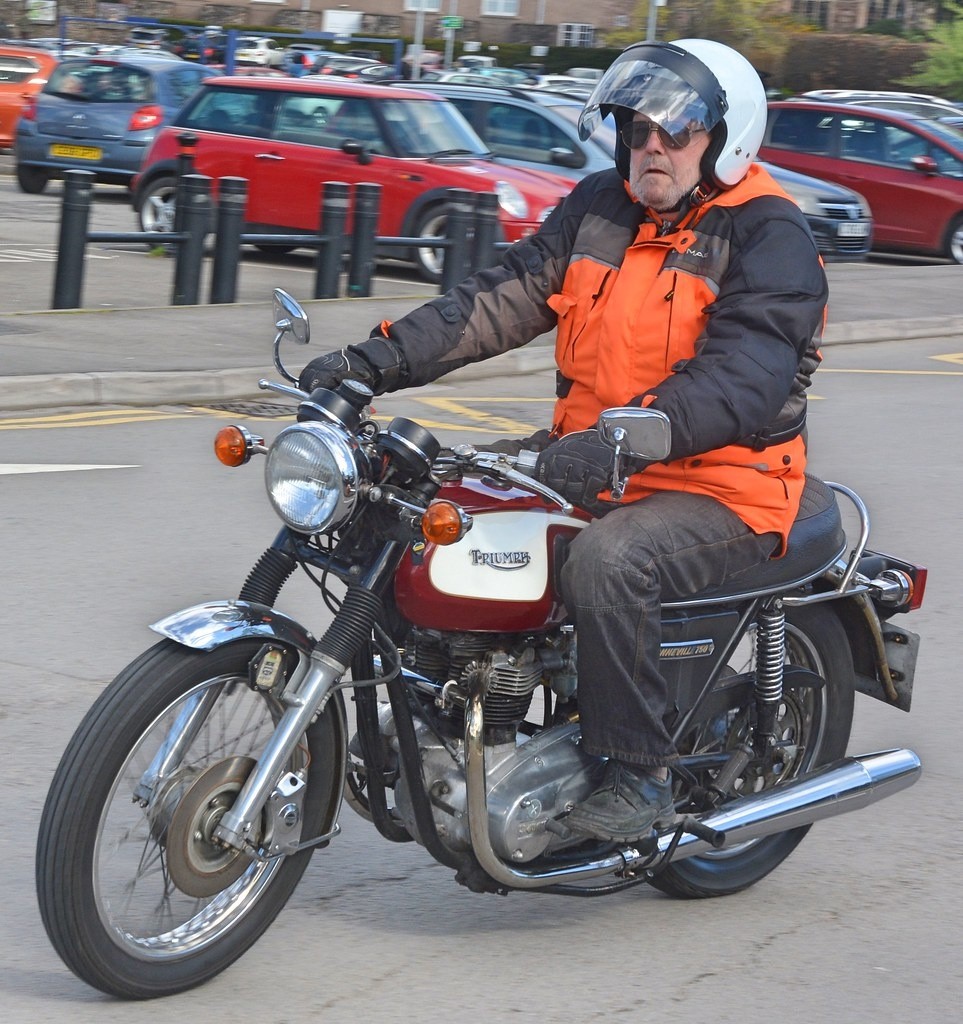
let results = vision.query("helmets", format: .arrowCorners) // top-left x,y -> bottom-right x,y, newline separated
577,37 -> 769,190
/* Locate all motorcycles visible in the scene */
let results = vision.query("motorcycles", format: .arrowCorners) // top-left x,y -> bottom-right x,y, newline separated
35,287 -> 928,997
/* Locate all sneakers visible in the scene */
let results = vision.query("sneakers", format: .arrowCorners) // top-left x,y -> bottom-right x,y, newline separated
562,755 -> 676,843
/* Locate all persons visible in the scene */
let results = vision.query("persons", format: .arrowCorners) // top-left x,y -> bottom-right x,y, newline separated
295,32 -> 832,844
399,54 -> 425,82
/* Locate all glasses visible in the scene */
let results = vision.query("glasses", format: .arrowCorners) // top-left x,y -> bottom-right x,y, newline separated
619,120 -> 708,150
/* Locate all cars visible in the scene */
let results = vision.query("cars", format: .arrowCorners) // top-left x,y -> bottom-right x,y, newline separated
279,43 -> 324,77
758,88 -> 963,266
1,35 -> 226,199
333,77 -> 872,264
234,36 -> 285,70
131,76 -> 582,279
288,50 -> 605,99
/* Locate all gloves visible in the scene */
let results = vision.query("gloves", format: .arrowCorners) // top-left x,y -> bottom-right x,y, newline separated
532,429 -> 625,507
298,349 -> 374,406
